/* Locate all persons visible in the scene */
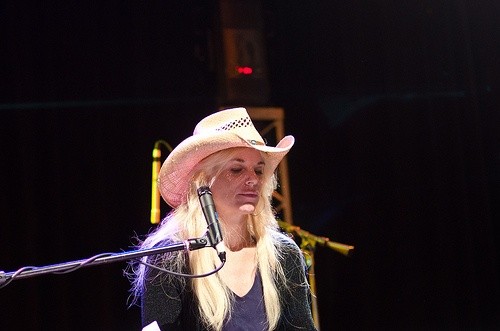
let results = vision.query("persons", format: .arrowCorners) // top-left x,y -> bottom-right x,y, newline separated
121,107 -> 319,331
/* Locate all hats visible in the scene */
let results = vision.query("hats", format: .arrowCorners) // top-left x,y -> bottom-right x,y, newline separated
158,105 -> 296,209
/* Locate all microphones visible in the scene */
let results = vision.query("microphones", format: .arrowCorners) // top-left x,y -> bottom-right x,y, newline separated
150,148 -> 162,224
197,186 -> 227,262
